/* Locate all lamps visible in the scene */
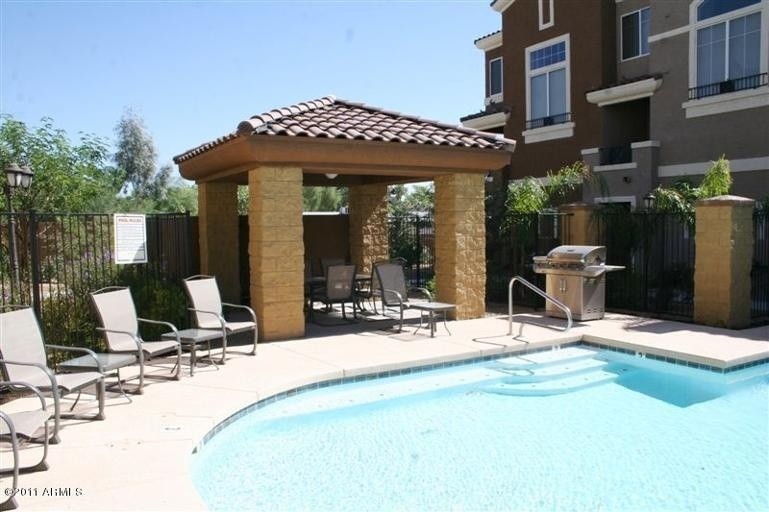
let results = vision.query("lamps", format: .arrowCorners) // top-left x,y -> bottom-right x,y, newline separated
388,188 -> 399,198
325,173 -> 339,180
486,171 -> 494,183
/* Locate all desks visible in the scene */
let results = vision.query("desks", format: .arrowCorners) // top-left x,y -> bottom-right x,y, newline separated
410,302 -> 457,339
56,351 -> 137,411
160,328 -> 224,377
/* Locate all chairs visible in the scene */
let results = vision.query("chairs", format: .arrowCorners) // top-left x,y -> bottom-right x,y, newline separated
89,284 -> 183,394
0,305 -> 117,446
183,274 -> 259,366
304,254 -> 437,335
1,378 -> 52,510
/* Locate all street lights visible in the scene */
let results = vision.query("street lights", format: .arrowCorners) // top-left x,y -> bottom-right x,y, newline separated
643,190 -> 655,212
1,162 -> 36,304
